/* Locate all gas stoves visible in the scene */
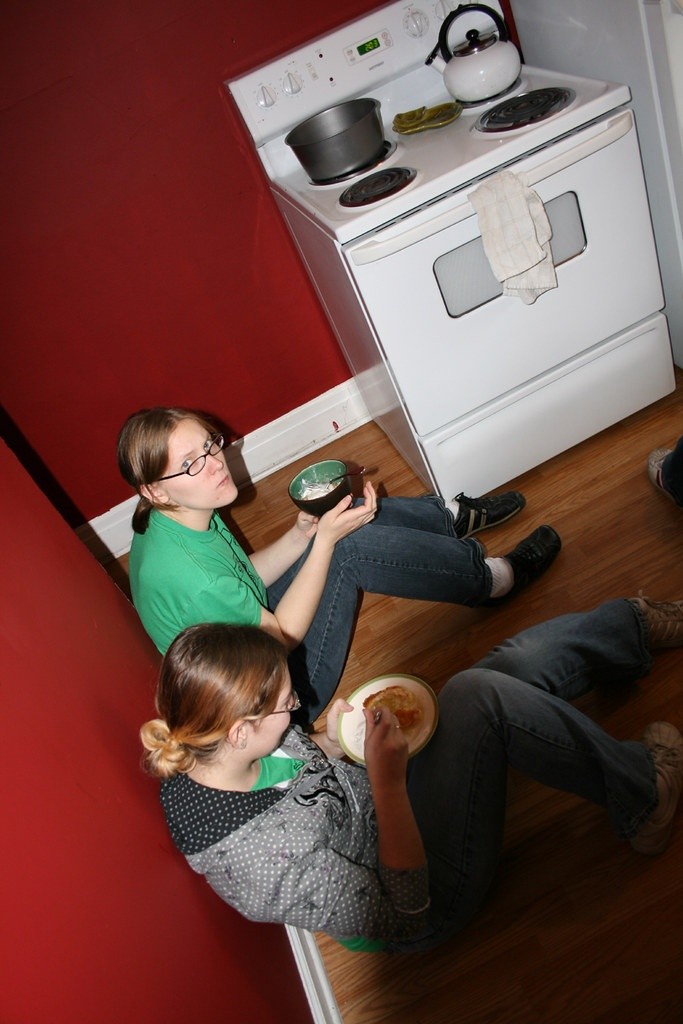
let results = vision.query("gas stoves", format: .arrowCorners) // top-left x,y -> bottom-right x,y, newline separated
223,0 -> 632,244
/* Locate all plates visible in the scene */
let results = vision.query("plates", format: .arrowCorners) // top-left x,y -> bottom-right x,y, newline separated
336,674 -> 439,765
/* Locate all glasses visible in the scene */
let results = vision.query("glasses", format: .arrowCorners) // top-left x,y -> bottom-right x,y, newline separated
270,688 -> 302,715
153,432 -> 224,482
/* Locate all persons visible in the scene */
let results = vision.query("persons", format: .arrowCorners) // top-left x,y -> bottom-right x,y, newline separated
138,591 -> 683,952
647,433 -> 683,511
117,409 -> 560,732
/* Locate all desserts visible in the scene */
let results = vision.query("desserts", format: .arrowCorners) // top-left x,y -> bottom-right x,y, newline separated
364,686 -> 422,739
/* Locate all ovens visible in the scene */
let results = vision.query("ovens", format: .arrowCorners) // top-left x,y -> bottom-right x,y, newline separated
341,108 -> 677,501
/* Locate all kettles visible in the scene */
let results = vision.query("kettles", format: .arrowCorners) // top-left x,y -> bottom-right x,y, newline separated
424,3 -> 521,103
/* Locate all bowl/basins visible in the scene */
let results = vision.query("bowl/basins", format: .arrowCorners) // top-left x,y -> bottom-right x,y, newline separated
288,459 -> 352,518
284,98 -> 385,183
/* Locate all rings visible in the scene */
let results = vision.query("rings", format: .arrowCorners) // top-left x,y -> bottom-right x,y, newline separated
389,725 -> 402,731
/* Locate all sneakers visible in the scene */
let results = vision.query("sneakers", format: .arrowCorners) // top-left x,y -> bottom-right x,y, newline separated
647,448 -> 678,506
628,589 -> 683,649
629,720 -> 683,855
452,490 -> 526,540
485,525 -> 561,608
